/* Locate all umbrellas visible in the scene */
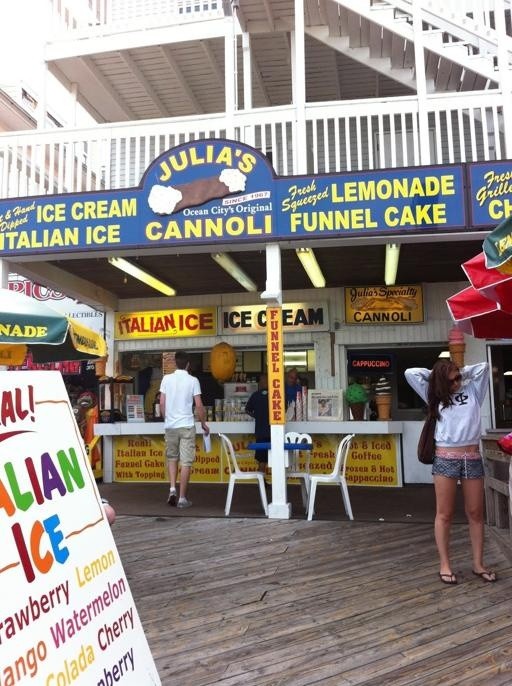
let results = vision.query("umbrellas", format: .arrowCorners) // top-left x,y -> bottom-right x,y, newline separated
446,213 -> 512,339
0,288 -> 107,364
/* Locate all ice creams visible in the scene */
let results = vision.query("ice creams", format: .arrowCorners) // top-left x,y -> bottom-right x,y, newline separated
447,325 -> 465,367
344,382 -> 369,420
374,375 -> 393,419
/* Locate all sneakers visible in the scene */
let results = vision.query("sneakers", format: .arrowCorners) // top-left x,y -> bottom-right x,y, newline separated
177,500 -> 192,508
167,491 -> 178,505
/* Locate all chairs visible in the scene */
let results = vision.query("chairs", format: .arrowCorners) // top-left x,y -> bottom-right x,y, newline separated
303,434 -> 357,522
218,434 -> 271,519
280,429 -> 315,516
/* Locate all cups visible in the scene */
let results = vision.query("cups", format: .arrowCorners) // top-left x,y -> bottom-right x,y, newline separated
201,398 -> 259,422
294,384 -> 307,420
227,370 -> 257,384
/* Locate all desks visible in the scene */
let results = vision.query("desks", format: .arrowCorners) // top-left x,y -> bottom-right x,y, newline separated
249,439 -> 317,518
478,428 -> 511,532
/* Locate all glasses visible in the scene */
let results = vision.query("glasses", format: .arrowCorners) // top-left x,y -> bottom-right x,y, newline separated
449,374 -> 461,385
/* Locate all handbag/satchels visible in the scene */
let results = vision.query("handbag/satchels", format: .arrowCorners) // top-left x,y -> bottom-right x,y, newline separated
417,410 -> 438,463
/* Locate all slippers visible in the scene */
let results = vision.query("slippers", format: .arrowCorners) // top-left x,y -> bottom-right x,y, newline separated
438,571 -> 457,584
472,569 -> 496,582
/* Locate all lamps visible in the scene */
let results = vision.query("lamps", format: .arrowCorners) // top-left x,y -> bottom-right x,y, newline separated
109,255 -> 175,297
212,252 -> 258,292
383,241 -> 401,286
295,247 -> 326,290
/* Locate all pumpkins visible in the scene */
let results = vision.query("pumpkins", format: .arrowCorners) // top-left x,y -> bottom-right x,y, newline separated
211,342 -> 235,380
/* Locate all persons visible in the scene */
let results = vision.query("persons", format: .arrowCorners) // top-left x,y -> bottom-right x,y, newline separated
246,376 -> 270,472
159,351 -> 210,508
100,496 -> 115,527
284,368 -> 302,408
403,359 -> 497,585
